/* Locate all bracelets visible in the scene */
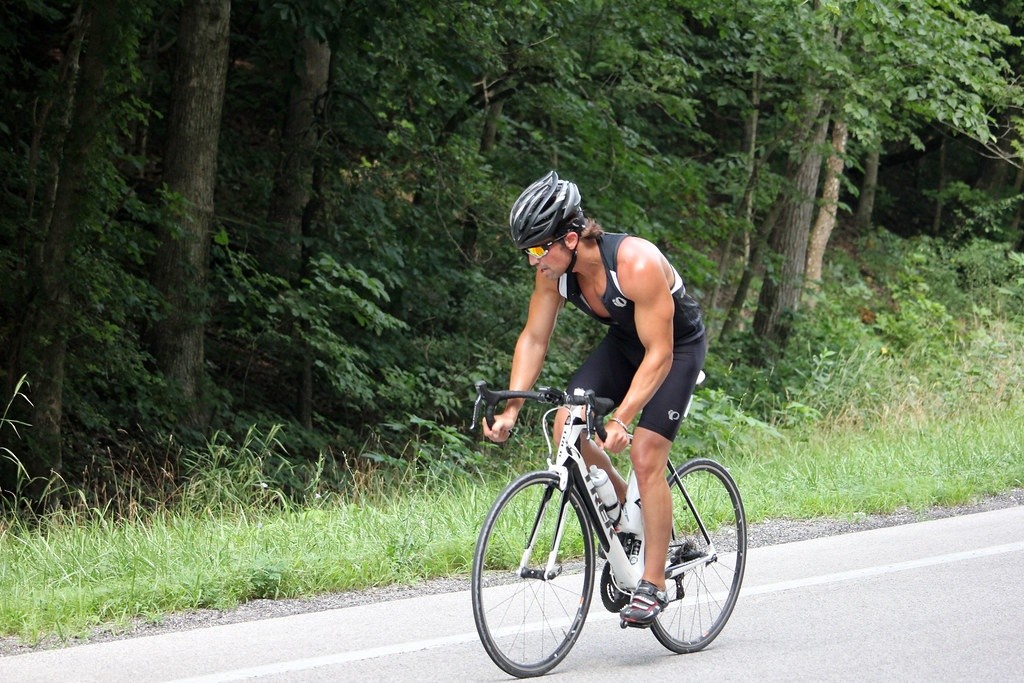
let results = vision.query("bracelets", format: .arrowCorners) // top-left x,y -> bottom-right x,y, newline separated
610,418 -> 628,432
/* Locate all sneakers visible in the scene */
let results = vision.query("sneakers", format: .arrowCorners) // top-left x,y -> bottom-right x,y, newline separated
597,502 -> 629,560
619,578 -> 671,626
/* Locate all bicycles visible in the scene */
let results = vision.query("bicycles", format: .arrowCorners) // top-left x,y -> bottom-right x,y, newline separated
469,380 -> 748,678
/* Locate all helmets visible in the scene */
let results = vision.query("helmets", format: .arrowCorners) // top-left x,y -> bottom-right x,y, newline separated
510,170 -> 586,250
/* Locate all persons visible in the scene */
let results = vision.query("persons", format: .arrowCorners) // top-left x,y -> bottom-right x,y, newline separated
482,170 -> 706,624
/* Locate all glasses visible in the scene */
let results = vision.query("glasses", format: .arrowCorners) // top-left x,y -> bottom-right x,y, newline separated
521,228 -> 578,260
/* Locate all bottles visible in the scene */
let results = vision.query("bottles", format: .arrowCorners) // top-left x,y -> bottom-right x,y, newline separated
622,469 -> 643,536
589,465 -> 628,534
